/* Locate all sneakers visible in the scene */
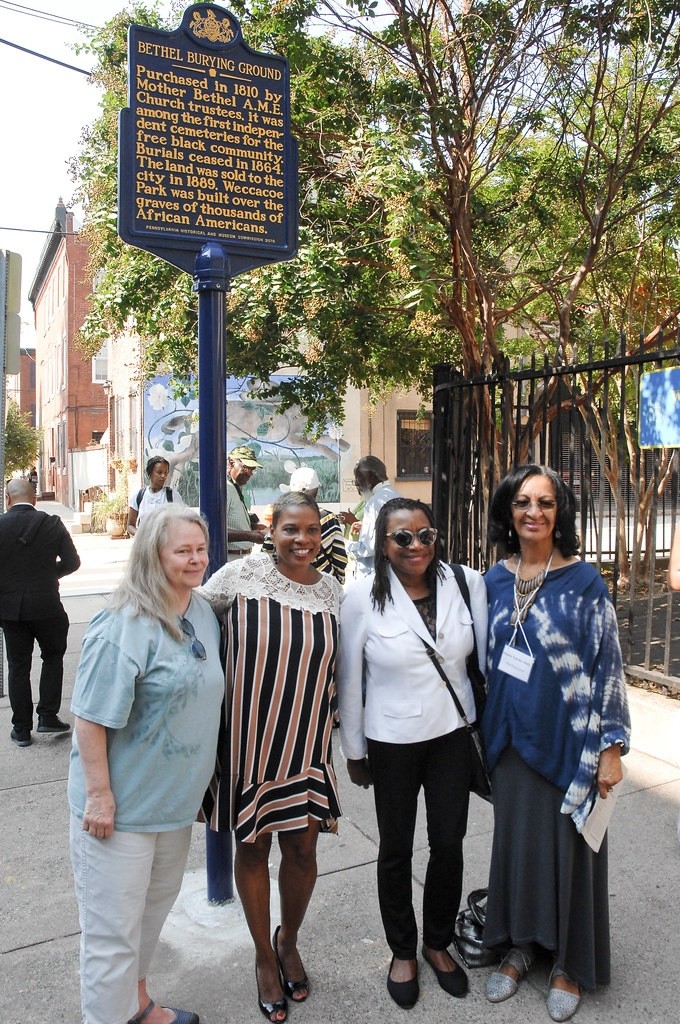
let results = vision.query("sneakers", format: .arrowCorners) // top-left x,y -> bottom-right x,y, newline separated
36,717 -> 70,732
11,728 -> 30,746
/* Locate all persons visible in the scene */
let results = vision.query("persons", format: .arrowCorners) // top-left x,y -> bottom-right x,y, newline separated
28,467 -> 38,496
127,455 -> 182,536
68,503 -> 227,1024
226,445 -> 269,565
262,467 -> 348,586
337,492 -> 365,543
0,480 -> 81,746
340,455 -> 404,587
484,464 -> 631,1021
194,493 -> 346,1024
335,497 -> 488,1010
666,520 -> 680,594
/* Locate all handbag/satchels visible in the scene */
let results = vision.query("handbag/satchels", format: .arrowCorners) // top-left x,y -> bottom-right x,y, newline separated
453,886 -> 505,968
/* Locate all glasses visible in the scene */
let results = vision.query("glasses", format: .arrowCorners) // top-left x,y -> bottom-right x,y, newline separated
180,618 -> 207,660
385,527 -> 438,548
511,498 -> 557,512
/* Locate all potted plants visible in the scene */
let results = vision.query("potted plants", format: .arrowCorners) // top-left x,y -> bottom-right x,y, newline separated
89,494 -> 127,540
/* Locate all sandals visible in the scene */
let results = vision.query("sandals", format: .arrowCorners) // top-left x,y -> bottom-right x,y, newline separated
255,951 -> 289,1024
273,925 -> 311,1001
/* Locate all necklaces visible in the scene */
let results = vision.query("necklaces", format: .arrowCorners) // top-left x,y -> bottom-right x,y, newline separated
510,548 -> 554,626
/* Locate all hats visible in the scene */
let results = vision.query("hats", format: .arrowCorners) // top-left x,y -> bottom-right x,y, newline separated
291,468 -> 322,492
228,446 -> 263,468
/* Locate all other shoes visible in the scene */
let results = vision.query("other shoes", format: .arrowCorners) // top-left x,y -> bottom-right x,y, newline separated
129,998 -> 199,1024
422,950 -> 468,998
387,953 -> 420,1010
484,948 -> 532,1002
546,963 -> 580,1021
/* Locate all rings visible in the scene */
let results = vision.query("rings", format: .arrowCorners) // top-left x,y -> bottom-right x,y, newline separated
607,788 -> 613,792
